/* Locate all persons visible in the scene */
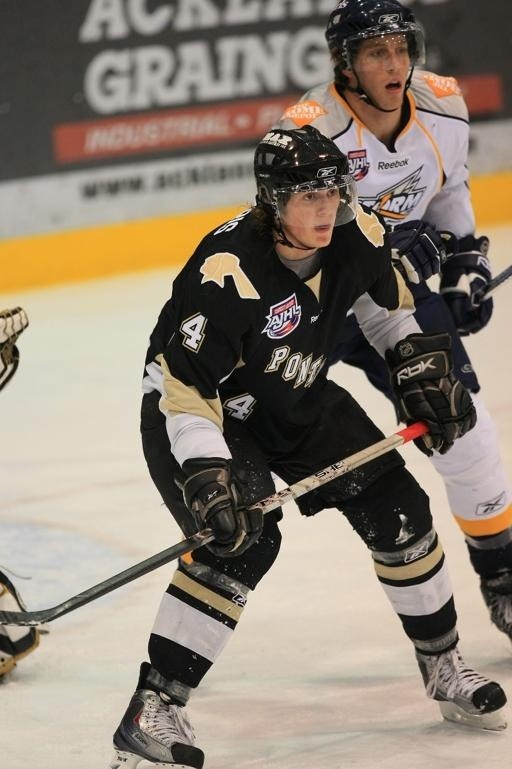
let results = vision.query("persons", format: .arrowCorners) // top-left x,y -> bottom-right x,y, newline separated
108,124 -> 508,769
266,0 -> 512,638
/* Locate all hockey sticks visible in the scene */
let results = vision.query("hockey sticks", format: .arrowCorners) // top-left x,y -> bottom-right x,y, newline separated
0,422 -> 428,626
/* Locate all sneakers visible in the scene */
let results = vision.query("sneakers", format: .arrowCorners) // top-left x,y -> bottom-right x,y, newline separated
476,574 -> 512,642
413,648 -> 506,717
108,658 -> 211,767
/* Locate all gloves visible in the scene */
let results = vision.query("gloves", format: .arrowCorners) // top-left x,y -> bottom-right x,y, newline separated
382,334 -> 479,459
433,228 -> 498,337
168,451 -> 266,563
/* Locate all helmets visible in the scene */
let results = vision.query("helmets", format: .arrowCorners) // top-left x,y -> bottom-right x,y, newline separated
251,123 -> 353,229
322,0 -> 427,75
